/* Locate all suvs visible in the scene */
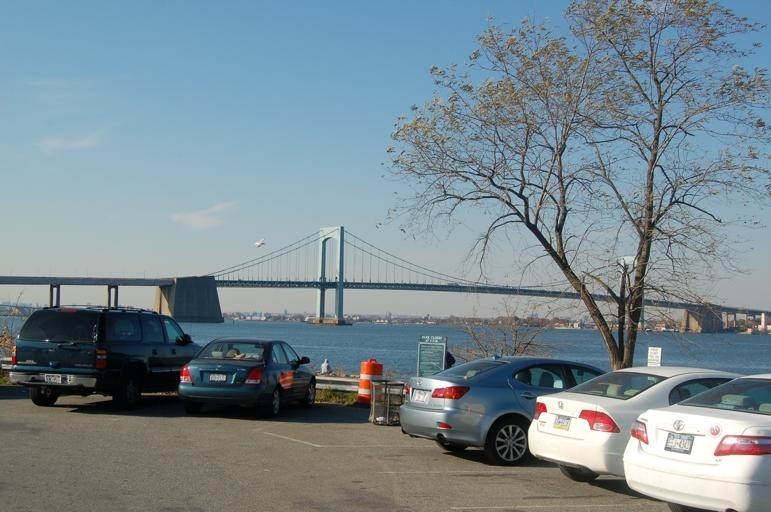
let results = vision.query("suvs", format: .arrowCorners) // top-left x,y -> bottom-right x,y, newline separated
9,304 -> 202,410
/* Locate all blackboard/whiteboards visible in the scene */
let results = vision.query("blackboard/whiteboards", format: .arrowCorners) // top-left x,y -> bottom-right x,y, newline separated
417,334 -> 447,377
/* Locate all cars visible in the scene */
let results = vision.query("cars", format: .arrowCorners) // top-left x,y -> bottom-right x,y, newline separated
398,354 -> 608,468
623,374 -> 771,512
178,336 -> 317,415
527,365 -> 743,482
